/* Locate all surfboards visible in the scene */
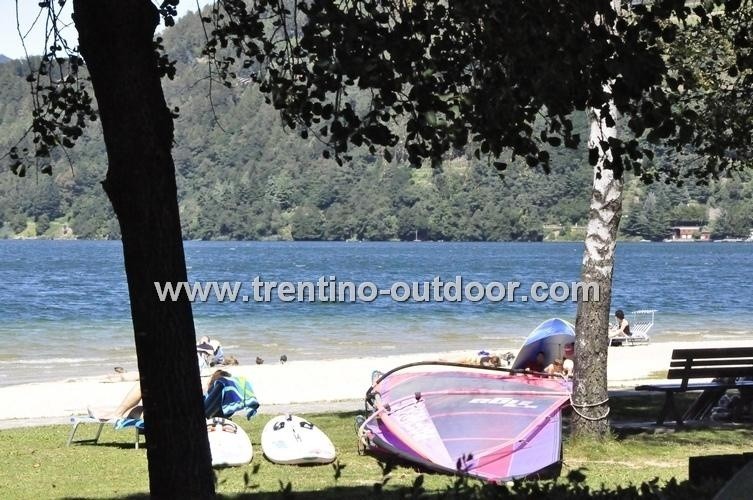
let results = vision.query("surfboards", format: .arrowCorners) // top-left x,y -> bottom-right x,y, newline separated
261,413 -> 336,464
207,417 -> 253,469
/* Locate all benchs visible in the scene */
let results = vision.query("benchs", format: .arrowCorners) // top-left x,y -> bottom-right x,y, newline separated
635,347 -> 753,434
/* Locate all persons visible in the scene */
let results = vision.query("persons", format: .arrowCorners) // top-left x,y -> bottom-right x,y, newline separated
562,342 -> 573,380
522,351 -> 546,379
85,369 -> 231,423
541,358 -> 561,381
609,310 -> 633,340
199,335 -> 287,365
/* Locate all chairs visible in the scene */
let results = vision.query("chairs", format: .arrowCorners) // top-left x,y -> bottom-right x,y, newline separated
608,308 -> 659,346
67,375 -> 260,450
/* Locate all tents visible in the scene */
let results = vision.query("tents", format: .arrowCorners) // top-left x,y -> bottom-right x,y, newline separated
508,317 -> 576,380
